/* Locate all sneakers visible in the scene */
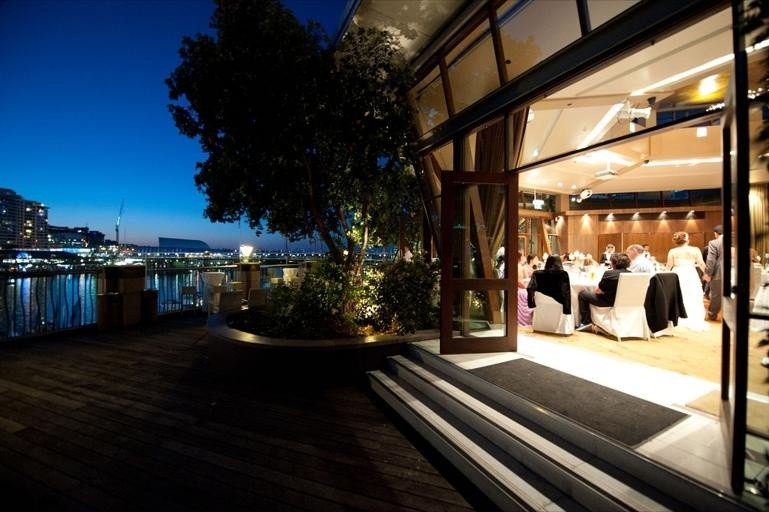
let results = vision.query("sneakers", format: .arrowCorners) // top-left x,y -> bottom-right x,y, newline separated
576,322 -> 592,330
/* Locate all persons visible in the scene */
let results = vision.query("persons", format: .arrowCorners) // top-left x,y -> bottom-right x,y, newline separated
703,246 -> 711,300
498,243 -> 656,332
703,224 -> 724,320
666,231 -> 711,331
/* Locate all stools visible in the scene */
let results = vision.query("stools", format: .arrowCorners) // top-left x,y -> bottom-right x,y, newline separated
180,286 -> 197,310
287,277 -> 301,282
272,277 -> 284,284
230,281 -> 246,289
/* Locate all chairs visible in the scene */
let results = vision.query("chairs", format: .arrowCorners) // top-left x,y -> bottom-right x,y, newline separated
242,287 -> 271,309
506,259 -> 769,385
207,289 -> 241,324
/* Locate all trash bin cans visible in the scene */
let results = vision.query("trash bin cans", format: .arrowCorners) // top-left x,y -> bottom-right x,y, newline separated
143,288 -> 160,317
94,291 -> 122,325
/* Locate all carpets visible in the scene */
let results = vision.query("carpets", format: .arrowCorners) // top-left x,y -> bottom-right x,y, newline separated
686,380 -> 768,437
466,357 -> 692,453
